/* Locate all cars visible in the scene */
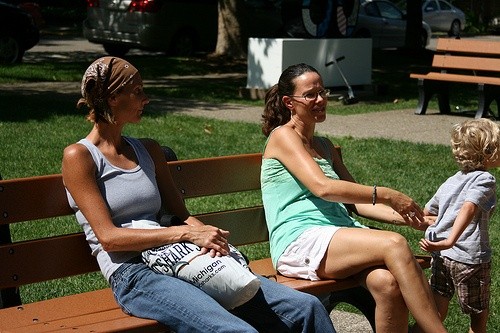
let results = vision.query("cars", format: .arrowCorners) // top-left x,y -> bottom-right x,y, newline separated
386,0 -> 469,38
0,0 -> 45,68
287,0 -> 433,55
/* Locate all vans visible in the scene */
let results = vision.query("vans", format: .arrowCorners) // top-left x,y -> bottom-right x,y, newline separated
80,0 -> 282,57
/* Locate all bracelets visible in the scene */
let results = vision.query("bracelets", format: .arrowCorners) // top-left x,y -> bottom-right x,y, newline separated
372,185 -> 376,205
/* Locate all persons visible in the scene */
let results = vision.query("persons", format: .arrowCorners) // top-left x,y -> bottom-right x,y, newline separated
259,63 -> 446,333
62,56 -> 336,333
409,117 -> 500,333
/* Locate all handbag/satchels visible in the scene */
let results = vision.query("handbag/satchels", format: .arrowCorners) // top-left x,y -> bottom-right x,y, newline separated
121,219 -> 261,312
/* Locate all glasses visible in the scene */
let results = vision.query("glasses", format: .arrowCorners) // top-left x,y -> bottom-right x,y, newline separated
290,88 -> 331,101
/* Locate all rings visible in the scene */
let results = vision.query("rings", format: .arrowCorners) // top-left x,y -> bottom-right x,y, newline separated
410,212 -> 415,217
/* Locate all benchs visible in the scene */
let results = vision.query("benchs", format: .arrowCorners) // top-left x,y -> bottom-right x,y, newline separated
1,147 -> 431,333
409,39 -> 500,119
